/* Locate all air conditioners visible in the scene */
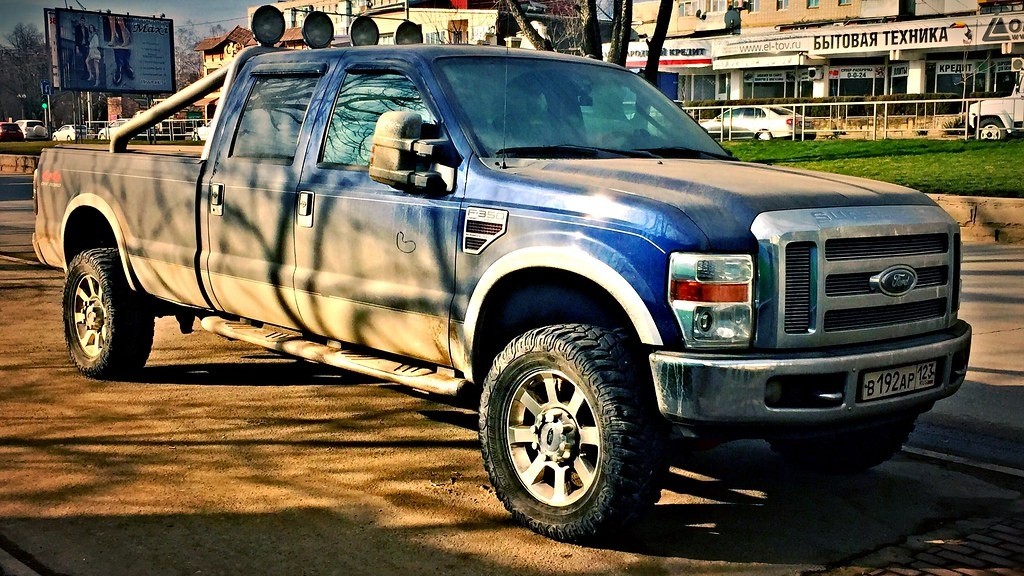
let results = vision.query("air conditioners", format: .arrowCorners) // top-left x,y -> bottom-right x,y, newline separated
1011,58 -> 1024,72
808,68 -> 822,79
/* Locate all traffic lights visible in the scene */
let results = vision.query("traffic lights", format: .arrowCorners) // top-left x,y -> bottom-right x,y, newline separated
41,94 -> 48,109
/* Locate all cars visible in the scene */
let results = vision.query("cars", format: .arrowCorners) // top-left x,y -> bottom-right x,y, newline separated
0,122 -> 24,142
699,105 -> 817,140
192,120 -> 211,142
98,118 -> 136,140
52,124 -> 87,141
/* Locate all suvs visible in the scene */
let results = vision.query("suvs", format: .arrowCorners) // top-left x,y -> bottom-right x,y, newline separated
969,74 -> 1024,141
14,120 -> 49,140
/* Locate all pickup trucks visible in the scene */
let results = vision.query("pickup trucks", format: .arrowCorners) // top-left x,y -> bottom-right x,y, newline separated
31,2 -> 973,545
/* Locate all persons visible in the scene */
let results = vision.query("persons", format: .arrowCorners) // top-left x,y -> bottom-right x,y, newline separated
111,48 -> 136,87
108,16 -> 133,47
72,15 -> 101,90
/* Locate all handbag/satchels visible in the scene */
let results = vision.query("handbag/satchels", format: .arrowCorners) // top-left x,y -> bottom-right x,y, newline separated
94,50 -> 100,59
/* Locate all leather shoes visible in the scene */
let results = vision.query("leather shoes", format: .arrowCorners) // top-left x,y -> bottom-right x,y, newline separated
113,66 -> 122,85
124,65 -> 136,79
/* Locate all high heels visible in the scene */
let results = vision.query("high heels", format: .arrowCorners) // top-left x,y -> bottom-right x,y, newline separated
121,29 -> 132,47
108,32 -> 119,46
